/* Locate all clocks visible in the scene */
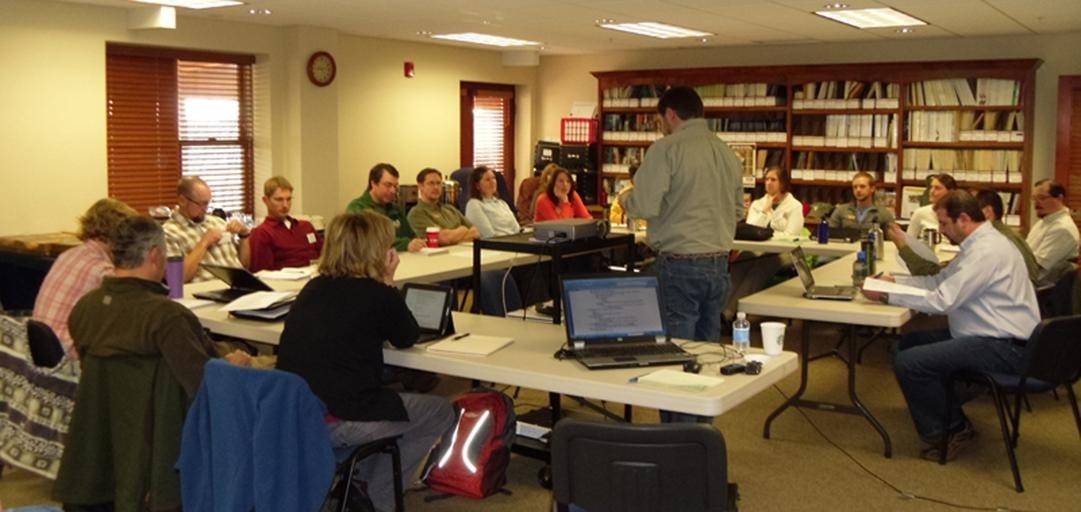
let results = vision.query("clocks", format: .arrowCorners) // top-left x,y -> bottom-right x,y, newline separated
306,51 -> 337,86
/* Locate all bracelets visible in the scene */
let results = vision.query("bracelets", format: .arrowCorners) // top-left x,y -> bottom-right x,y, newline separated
878,292 -> 889,302
239,233 -> 249,238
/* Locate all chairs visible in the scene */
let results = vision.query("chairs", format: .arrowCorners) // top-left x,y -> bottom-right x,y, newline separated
1065,254 -> 1079,266
551,417 -> 740,510
81,349 -> 189,510
182,359 -> 405,509
1035,269 -> 1073,319
2,315 -> 84,473
403,200 -> 458,221
936,315 -> 1080,493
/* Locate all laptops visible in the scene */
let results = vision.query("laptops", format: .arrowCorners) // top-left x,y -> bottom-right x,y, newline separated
790,246 -> 857,301
558,271 -> 697,370
191,263 -> 276,304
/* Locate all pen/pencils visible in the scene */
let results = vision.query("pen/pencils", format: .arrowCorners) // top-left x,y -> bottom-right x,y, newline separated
873,271 -> 884,279
452,332 -> 470,341
416,233 -> 429,247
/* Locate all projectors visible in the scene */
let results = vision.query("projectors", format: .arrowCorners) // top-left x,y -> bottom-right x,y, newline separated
531,218 -> 610,242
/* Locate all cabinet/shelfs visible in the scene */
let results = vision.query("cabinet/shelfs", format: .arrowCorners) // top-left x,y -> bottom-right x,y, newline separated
589,58 -> 1042,241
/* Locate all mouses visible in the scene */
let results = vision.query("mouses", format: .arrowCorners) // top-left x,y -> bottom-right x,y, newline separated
744,361 -> 763,375
683,360 -> 702,374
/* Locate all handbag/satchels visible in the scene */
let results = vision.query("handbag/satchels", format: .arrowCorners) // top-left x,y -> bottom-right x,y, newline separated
734,223 -> 773,241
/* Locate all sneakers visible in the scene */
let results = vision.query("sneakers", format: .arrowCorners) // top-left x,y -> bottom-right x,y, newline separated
921,422 -> 978,462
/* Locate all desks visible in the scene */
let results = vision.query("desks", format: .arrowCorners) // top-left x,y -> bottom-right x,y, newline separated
167,218 -> 960,492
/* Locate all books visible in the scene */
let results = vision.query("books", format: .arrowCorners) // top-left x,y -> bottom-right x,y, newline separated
223,290 -> 300,321
603,78 -> 1023,226
428,332 -> 517,359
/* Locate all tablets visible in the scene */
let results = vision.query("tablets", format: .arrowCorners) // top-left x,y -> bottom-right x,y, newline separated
400,282 -> 454,345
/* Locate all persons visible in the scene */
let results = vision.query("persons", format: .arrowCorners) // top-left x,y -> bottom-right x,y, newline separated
718,165 -> 806,324
855,186 -> 1043,462
406,170 -> 523,318
1025,179 -> 1081,273
28,197 -> 139,364
248,177 -> 324,274
535,171 -> 604,278
67,214 -> 278,401
344,164 -> 429,253
156,176 -> 252,284
464,166 -> 553,306
617,86 -> 744,425
529,163 -> 562,217
888,189 -> 1040,291
278,210 -> 455,511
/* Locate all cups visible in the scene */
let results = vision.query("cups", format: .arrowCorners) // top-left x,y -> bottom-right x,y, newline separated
426,226 -> 441,248
760,322 -> 786,353
924,228 -> 943,248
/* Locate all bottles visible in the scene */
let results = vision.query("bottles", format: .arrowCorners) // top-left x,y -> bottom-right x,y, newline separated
817,219 -> 829,244
733,312 -> 752,352
853,252 -> 869,284
869,223 -> 884,260
166,254 -> 184,299
861,240 -> 876,275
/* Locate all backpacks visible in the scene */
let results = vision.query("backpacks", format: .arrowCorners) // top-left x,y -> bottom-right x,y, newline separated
422,391 -> 515,499
